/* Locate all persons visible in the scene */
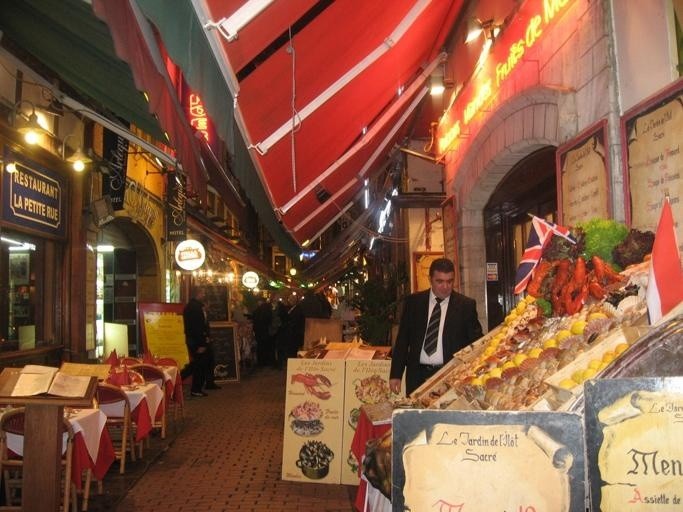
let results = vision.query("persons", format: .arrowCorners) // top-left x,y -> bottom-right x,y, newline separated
179,286 -> 212,397
179,295 -> 223,390
254,289 -> 332,370
386,257 -> 486,398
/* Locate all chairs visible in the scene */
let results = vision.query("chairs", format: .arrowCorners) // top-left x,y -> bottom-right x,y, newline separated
0,356 -> 181,512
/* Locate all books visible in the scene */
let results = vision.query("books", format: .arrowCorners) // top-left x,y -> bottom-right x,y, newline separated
11,371 -> 92,399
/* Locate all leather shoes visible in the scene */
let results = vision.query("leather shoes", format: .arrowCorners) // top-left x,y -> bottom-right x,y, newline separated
204,381 -> 222,390
191,390 -> 209,397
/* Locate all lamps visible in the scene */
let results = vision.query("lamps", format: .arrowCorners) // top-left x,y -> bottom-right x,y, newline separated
62,133 -> 92,172
146,170 -> 183,187
462,15 -> 501,46
127,152 -> 165,168
11,99 -> 54,145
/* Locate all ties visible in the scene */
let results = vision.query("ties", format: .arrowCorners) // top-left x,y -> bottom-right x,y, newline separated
424,298 -> 444,357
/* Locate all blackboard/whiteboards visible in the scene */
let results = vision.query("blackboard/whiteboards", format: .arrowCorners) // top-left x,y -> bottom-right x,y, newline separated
192,283 -> 240,383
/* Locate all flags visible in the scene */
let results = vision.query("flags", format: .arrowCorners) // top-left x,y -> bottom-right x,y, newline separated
513,214 -> 579,295
644,198 -> 682,327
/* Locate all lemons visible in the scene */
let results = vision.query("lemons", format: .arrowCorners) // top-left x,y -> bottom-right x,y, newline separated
471,294 -> 630,391
